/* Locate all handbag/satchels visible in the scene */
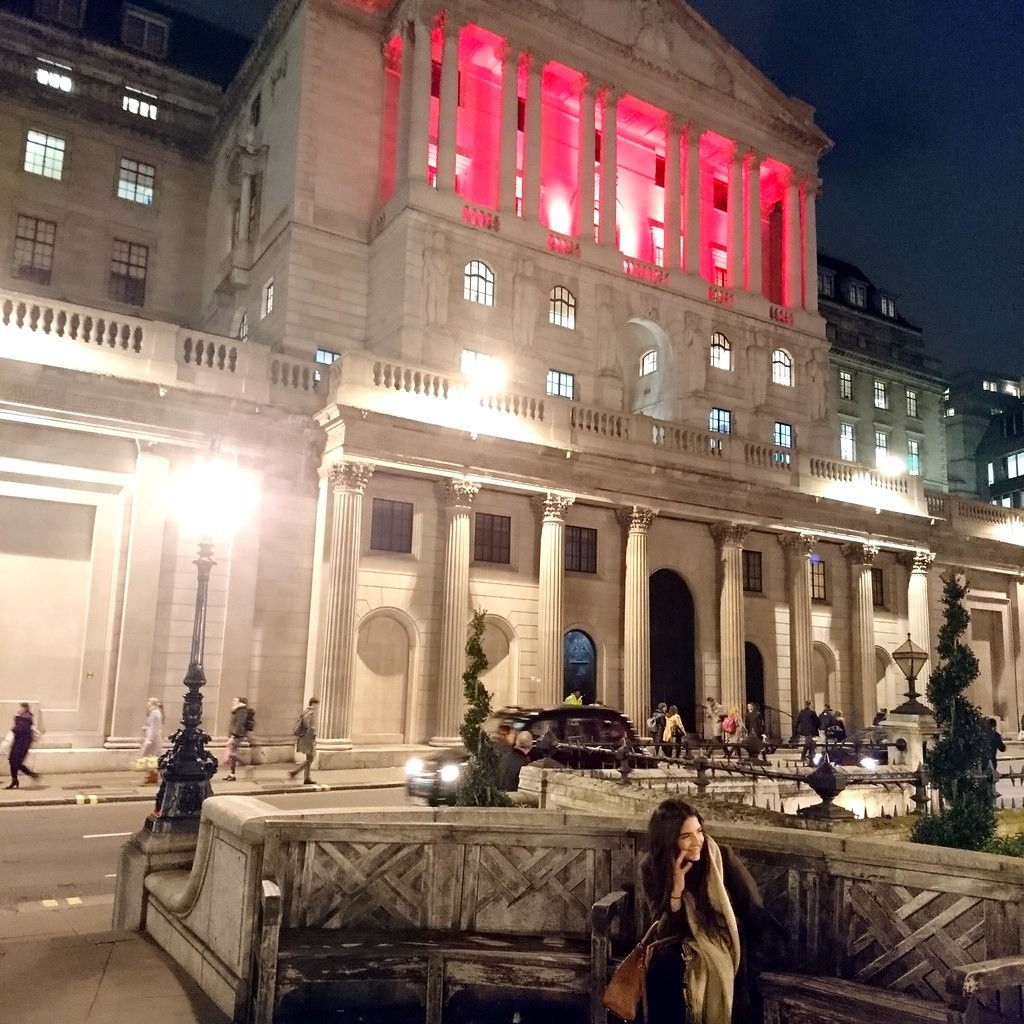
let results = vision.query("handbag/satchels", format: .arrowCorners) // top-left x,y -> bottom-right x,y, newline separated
825,727 -> 842,738
29,724 -> 40,742
761,740 -> 776,753
600,920 -> 661,1019
0,732 -> 14,756
134,754 -> 160,772
672,726 -> 685,738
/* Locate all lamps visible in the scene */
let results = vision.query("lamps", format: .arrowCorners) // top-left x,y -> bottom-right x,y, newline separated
891,632 -> 935,715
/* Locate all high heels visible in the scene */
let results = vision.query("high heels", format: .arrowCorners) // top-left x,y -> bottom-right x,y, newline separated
6,780 -> 19,789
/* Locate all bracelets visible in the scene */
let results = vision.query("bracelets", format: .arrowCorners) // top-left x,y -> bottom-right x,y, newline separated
671,896 -> 682,899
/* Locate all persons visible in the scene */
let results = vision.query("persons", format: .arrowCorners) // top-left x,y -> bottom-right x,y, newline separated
794,701 -> 848,761
140,697 -> 165,784
565,689 -> 583,736
651,703 -> 687,764
500,731 -> 533,792
986,718 -> 1006,769
489,723 -> 511,744
872,708 -> 888,726
222,696 -> 249,781
704,696 -> 766,759
1,703 -> 40,790
287,698 -> 321,785
638,798 -> 766,1024
588,699 -> 602,706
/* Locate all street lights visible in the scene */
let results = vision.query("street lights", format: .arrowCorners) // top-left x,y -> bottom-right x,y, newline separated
144,452 -> 263,834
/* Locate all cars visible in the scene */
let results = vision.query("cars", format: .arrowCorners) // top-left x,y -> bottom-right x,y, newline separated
817,729 -> 893,771
408,707 -> 647,802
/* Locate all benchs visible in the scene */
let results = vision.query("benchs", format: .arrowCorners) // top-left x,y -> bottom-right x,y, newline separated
255,828 -> 1024,1024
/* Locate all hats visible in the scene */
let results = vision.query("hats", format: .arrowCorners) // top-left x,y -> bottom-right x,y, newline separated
824,703 -> 833,710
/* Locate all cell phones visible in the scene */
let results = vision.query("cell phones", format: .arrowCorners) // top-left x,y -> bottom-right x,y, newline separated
674,843 -> 688,869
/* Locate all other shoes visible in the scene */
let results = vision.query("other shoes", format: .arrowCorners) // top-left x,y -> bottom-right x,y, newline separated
145,774 -> 157,783
223,775 -> 235,781
291,771 -> 297,777
704,753 -> 710,757
304,778 -> 315,784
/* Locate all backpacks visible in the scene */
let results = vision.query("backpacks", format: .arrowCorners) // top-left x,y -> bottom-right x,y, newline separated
645,714 -> 661,732
244,707 -> 255,731
721,717 -> 735,733
293,710 -> 311,738
